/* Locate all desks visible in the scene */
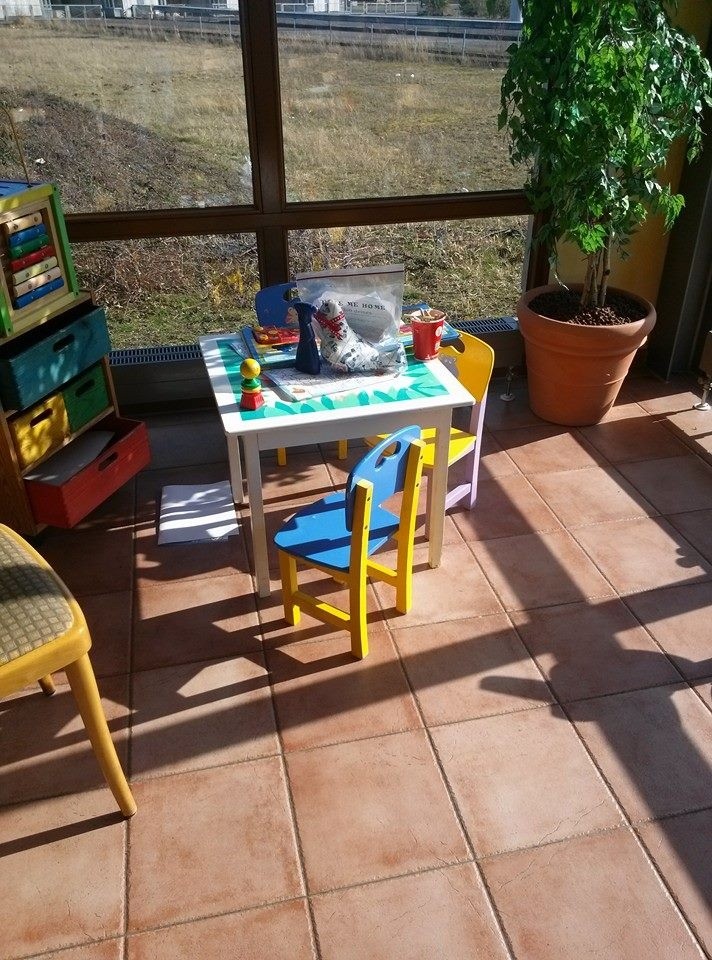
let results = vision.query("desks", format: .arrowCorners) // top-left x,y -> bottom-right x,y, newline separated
198,334 -> 476,599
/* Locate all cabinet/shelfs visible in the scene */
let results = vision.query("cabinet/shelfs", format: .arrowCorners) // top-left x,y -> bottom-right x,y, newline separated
0,180 -> 153,535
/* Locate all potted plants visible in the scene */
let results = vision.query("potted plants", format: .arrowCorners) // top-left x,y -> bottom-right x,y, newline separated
501,0 -> 712,426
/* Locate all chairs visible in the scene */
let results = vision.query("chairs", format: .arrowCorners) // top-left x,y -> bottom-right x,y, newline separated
364,331 -> 495,509
254,283 -> 348,466
273,425 -> 426,659
0,523 -> 137,817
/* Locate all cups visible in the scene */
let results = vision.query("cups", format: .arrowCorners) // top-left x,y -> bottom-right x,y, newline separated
409,309 -> 446,362
